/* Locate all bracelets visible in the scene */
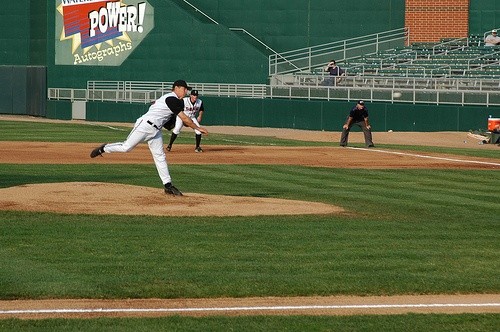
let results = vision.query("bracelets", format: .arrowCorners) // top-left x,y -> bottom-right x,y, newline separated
339,78 -> 341,80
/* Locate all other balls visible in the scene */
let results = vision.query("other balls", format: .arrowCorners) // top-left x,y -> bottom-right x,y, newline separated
393,91 -> 402,98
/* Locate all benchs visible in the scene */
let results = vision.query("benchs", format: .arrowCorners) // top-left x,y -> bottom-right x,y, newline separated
266,33 -> 499,107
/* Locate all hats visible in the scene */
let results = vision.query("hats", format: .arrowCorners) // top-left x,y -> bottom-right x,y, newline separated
357,100 -> 365,105
491,29 -> 497,33
191,90 -> 198,96
173,80 -> 192,90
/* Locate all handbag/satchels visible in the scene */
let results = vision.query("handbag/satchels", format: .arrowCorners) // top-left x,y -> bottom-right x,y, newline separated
336,77 -> 340,82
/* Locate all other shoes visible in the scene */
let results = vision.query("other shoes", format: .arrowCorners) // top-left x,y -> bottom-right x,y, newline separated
166,143 -> 172,152
195,147 -> 204,153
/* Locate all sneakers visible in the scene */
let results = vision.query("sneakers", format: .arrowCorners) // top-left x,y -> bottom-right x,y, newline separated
165,184 -> 183,196
90,145 -> 105,158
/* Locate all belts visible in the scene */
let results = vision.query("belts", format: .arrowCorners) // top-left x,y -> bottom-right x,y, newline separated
147,120 -> 159,130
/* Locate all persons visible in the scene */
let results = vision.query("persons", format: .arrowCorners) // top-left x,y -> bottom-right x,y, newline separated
340,100 -> 377,148
321,60 -> 344,90
166,90 -> 204,153
484,29 -> 500,46
90,80 -> 209,196
